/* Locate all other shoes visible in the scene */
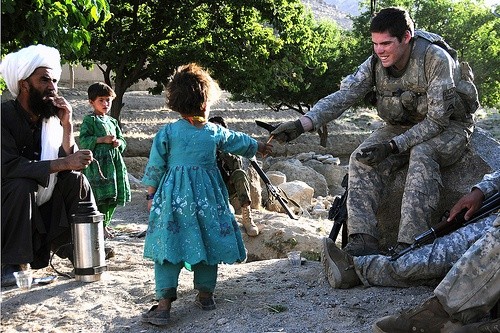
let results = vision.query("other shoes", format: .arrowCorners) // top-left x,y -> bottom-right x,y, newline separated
105,247 -> 114,260
1,264 -> 22,288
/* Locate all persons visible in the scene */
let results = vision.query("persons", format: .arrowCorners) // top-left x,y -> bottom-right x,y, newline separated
270,8 -> 476,259
79,82 -> 132,238
0,45 -> 93,287
209,116 -> 258,236
322,169 -> 500,333
142,62 -> 274,326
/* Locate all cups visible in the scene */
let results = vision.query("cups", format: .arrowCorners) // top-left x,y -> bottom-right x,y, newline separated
13,271 -> 33,290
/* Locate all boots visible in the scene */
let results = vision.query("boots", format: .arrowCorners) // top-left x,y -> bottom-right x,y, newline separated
242,205 -> 259,236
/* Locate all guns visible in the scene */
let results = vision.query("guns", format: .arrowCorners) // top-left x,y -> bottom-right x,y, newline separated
249,159 -> 304,221
388,192 -> 500,262
328,173 -> 348,249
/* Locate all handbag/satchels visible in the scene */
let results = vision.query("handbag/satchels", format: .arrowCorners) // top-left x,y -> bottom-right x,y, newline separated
413,31 -> 457,59
376,91 -> 428,125
455,61 -> 480,113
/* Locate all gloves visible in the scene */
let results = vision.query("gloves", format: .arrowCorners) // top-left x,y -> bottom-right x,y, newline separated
269,119 -> 305,145
356,142 -> 391,166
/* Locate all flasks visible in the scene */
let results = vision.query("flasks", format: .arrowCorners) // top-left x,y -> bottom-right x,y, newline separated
70,202 -> 107,282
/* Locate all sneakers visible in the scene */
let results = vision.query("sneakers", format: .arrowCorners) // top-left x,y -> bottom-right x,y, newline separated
372,297 -> 449,333
445,317 -> 500,333
342,234 -> 380,256
320,237 -> 360,289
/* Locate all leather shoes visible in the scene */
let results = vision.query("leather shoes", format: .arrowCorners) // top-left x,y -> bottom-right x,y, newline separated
194,292 -> 216,310
141,305 -> 170,325
